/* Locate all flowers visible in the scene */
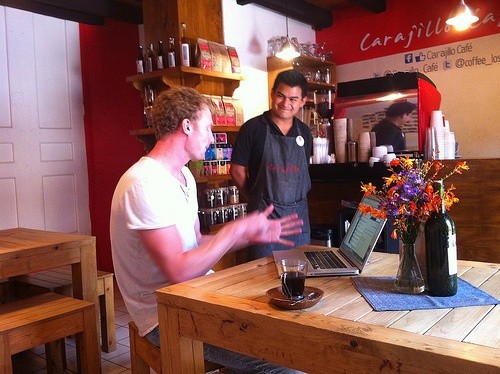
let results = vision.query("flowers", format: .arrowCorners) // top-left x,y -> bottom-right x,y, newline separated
358,151 -> 470,283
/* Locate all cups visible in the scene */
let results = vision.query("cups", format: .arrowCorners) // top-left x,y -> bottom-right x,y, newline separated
278,259 -> 309,301
358,132 -> 371,163
424,110 -> 455,161
333,118 -> 349,164
369,132 -> 376,151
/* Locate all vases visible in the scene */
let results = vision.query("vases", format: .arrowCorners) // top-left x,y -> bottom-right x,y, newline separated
393,243 -> 425,295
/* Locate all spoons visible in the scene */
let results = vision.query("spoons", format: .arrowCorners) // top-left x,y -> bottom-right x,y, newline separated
289,291 -> 320,306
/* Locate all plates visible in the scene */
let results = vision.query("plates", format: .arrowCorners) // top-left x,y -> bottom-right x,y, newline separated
265,285 -> 323,310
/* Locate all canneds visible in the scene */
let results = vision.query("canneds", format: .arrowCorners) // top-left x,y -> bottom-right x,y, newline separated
198,186 -> 247,232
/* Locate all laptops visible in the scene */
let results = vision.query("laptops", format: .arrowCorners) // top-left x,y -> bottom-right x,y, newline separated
271,194 -> 388,277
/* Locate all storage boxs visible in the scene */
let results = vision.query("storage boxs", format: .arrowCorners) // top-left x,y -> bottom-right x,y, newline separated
189,133 -> 233,176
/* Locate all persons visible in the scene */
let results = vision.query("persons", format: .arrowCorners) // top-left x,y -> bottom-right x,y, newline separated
109,87 -> 306,374
230,70 -> 313,262
372,102 -> 417,149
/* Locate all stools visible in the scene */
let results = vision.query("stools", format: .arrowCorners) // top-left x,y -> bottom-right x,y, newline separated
0,292 -> 102,374
311,224 -> 337,248
17,264 -> 116,353
128,320 -> 227,374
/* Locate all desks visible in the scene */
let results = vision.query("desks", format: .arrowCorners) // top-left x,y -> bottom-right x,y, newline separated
0,227 -> 103,374
156,245 -> 500,374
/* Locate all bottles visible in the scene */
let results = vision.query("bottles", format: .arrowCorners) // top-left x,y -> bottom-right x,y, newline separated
135,45 -> 145,75
145,43 -> 157,72
167,37 -> 176,68
268,37 -> 335,84
326,229 -> 333,247
179,22 -> 192,67
157,40 -> 166,69
195,185 -> 249,235
422,181 -> 460,297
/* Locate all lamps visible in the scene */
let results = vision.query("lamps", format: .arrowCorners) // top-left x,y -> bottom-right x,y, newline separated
445,0 -> 479,30
274,0 -> 302,60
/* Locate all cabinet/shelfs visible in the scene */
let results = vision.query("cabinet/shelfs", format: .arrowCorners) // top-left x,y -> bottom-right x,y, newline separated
127,64 -> 243,231
267,54 -> 337,127
